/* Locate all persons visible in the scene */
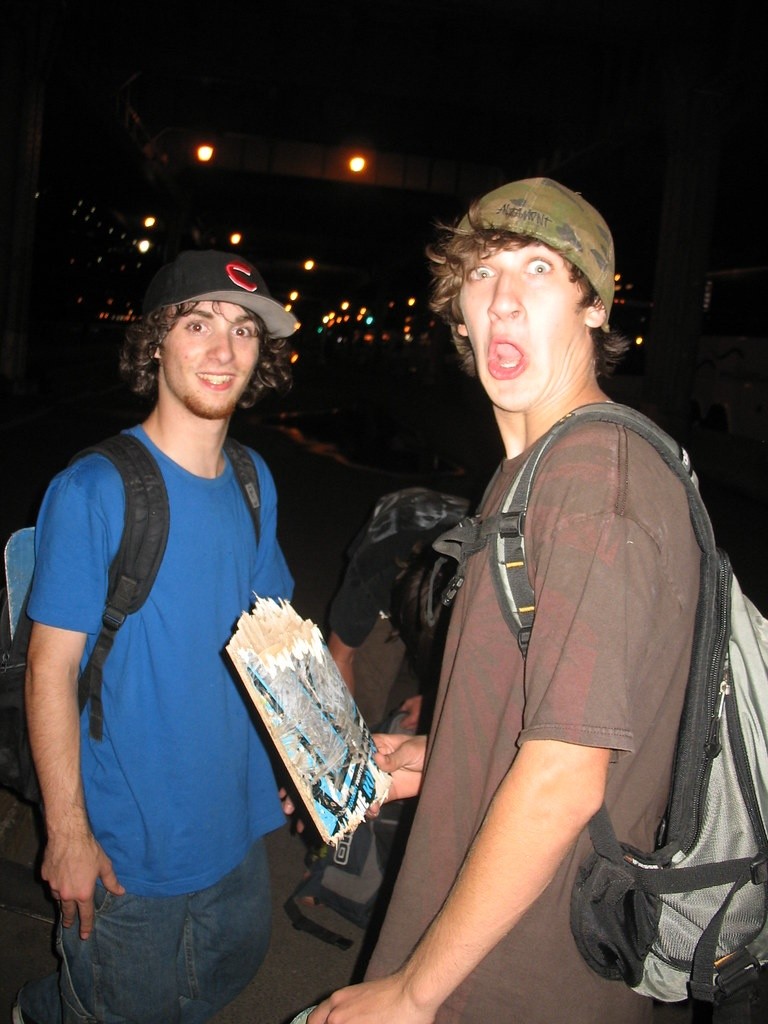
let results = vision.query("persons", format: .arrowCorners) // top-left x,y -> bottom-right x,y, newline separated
11,246 -> 296,1024
326,486 -> 475,736
293,177 -> 713,1024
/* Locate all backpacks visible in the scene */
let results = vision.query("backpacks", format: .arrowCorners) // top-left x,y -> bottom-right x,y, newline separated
489,402 -> 768,1002
0,435 -> 264,803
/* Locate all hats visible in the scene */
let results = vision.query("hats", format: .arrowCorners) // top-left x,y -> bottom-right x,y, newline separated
449,177 -> 615,330
143,250 -> 298,341
